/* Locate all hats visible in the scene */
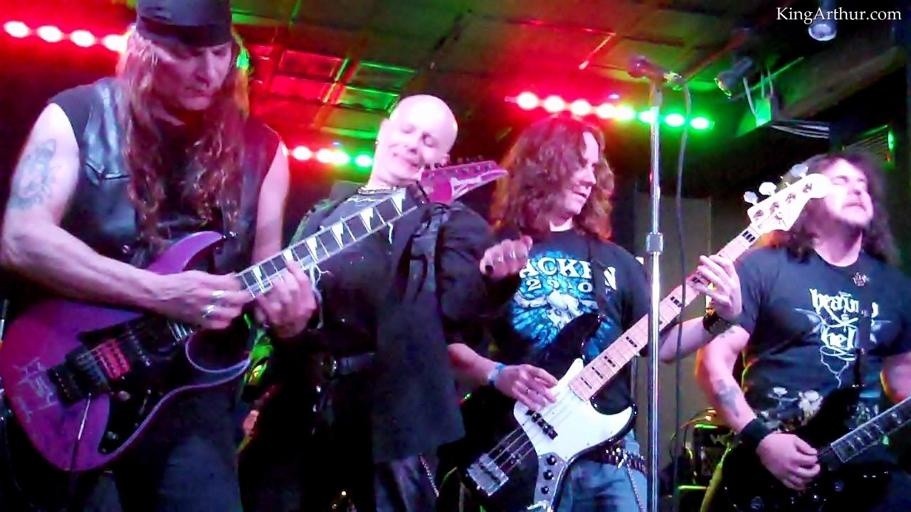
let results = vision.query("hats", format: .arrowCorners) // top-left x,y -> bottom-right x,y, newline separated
137,1 -> 231,45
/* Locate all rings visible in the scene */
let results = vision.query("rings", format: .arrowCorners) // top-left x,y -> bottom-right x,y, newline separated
210,289 -> 226,307
525,387 -> 530,394
199,305 -> 216,317
484,246 -> 526,272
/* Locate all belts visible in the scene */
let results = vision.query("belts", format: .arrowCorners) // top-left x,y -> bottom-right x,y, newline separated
317,354 -> 376,378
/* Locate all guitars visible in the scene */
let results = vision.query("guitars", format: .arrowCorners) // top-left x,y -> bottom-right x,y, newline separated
722,397 -> 911,512
1,156 -> 508,472
429,165 -> 828,512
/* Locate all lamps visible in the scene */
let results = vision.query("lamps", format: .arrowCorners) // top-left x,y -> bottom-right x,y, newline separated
713,55 -> 761,118
807,1 -> 838,44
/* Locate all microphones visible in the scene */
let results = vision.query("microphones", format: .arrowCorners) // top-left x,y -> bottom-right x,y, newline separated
625,54 -> 687,87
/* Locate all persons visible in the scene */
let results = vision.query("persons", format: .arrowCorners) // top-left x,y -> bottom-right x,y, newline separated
694,148 -> 911,511
0,0 -> 323,512
441,113 -> 743,512
237,93 -> 532,512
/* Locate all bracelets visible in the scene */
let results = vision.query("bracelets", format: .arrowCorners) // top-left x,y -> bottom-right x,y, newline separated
488,362 -> 505,388
737,419 -> 771,453
702,311 -> 735,339
